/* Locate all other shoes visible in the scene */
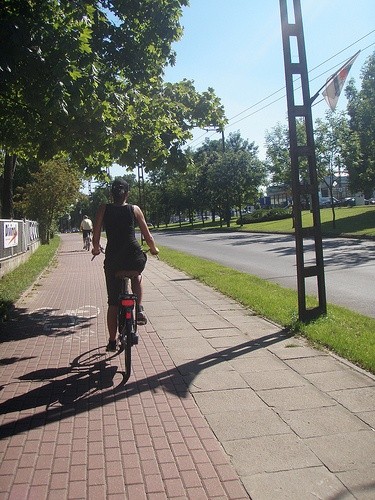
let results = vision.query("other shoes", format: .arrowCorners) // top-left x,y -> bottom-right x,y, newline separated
136,312 -> 147,324
106,343 -> 116,350
90,238 -> 92,242
83,246 -> 86,249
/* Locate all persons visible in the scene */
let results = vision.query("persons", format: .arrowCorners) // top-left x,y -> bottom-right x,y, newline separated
91,179 -> 160,352
80,215 -> 93,249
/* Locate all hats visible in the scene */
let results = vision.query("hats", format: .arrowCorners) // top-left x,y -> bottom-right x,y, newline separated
112,178 -> 129,194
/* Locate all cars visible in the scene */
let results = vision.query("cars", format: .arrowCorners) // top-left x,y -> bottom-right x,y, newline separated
319,196 -> 375,209
175,215 -> 220,223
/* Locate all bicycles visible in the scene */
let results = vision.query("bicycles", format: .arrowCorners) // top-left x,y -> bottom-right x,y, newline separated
91,244 -> 160,379
82,228 -> 93,251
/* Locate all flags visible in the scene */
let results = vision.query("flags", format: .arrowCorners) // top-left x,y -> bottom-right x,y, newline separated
321,50 -> 362,111
